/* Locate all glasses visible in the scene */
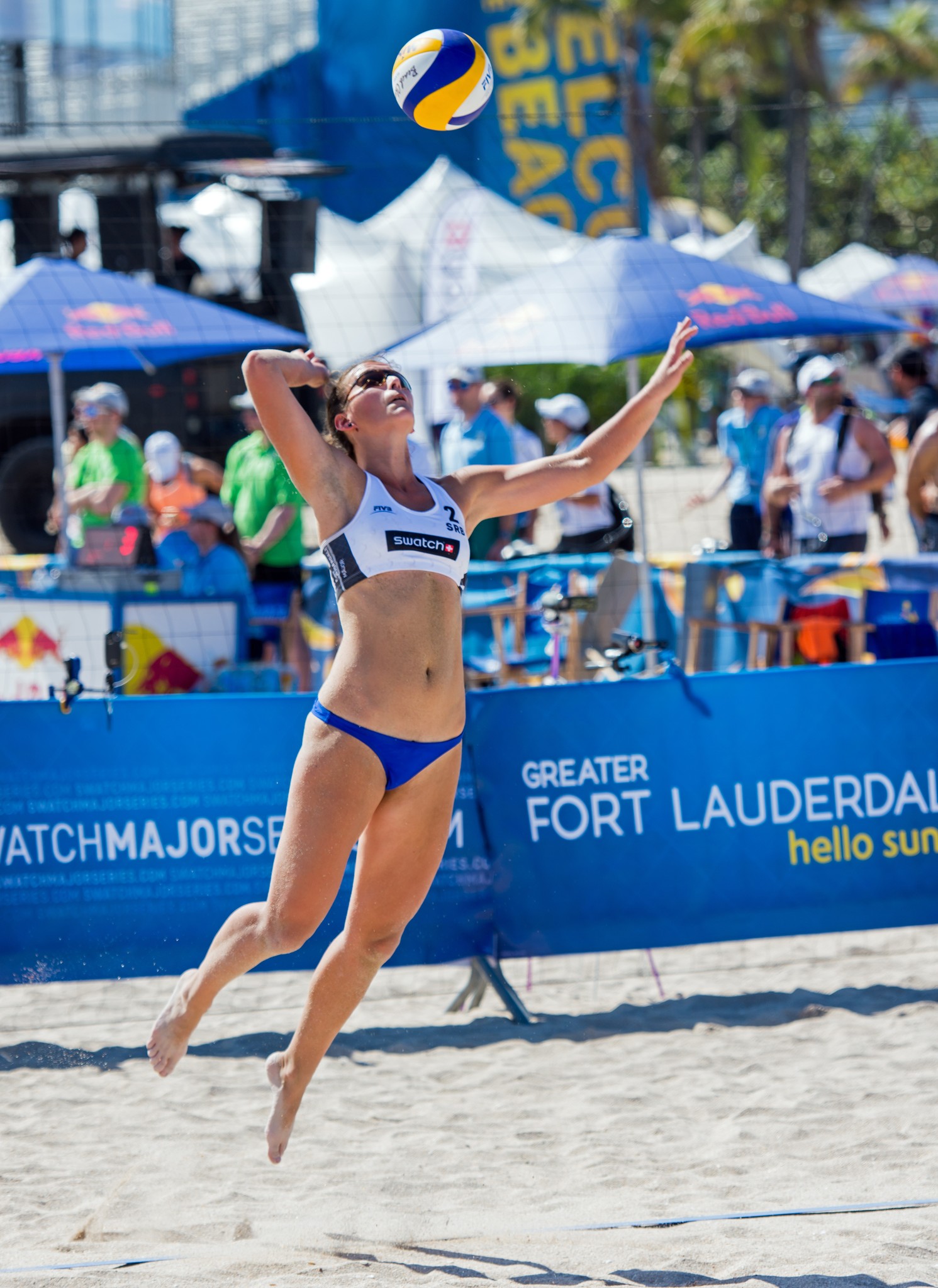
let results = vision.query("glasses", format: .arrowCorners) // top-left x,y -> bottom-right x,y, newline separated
449,382 -> 470,389
342,369 -> 413,404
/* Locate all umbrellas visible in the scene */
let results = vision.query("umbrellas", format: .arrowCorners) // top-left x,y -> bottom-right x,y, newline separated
0,186 -> 938,677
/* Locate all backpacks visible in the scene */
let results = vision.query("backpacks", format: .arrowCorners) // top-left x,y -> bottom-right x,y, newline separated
788,398 -> 888,537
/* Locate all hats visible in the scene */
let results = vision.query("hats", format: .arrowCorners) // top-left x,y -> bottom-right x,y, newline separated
534,393 -> 590,430
145,431 -> 181,484
77,381 -> 129,416
796,354 -> 841,395
733,368 -> 771,396
881,341 -> 927,372
447,365 -> 478,383
187,499 -> 232,528
231,389 -> 255,410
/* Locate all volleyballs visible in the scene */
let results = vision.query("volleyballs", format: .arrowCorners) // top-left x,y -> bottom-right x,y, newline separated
391,28 -> 494,130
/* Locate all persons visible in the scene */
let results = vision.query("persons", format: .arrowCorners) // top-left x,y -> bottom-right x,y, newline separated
145,315 -> 700,1163
64,232 -> 89,266
160,231 -> 207,299
39,339 -> 938,686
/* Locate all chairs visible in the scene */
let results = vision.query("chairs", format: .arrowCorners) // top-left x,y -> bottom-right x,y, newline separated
284,571 -> 878,691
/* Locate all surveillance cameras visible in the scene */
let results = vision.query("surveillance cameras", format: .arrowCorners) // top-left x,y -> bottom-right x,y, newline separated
609,630 -> 645,654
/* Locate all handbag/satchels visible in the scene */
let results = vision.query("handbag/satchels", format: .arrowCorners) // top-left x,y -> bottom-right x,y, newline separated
564,486 -> 635,554
